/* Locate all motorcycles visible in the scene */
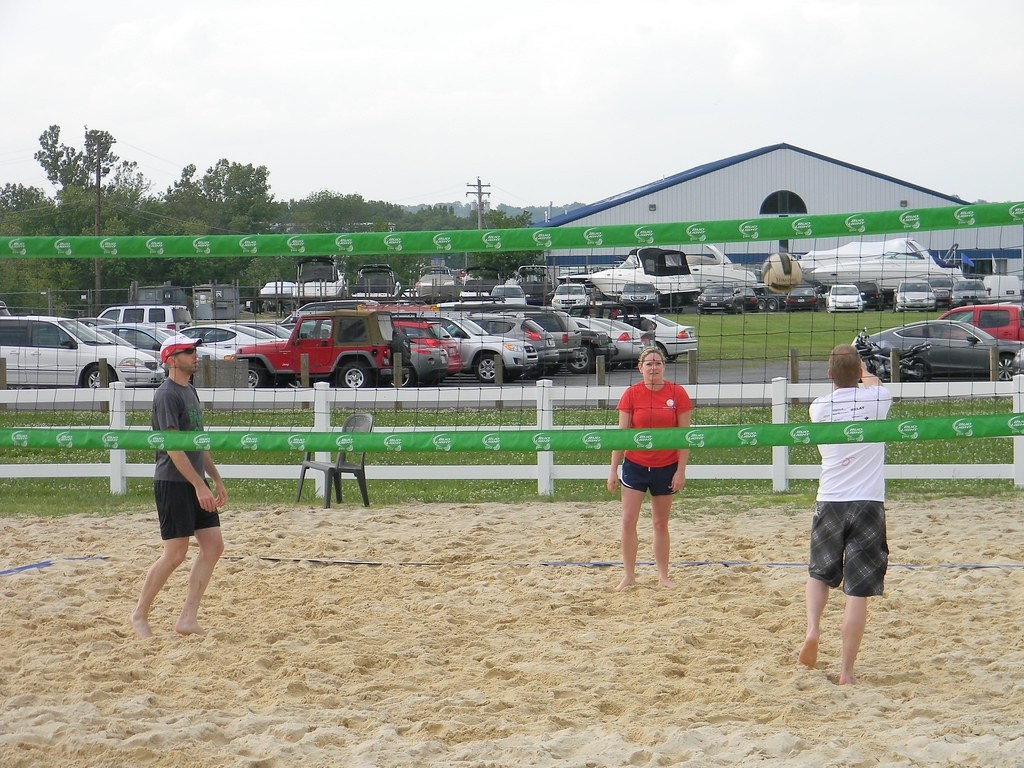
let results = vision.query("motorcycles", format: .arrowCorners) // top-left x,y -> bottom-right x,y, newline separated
850,323 -> 932,383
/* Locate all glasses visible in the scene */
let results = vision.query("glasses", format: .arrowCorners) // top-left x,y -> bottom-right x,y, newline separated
169,348 -> 196,356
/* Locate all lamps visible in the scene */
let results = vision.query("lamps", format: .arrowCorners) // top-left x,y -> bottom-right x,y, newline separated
649,204 -> 656,211
901,200 -> 908,207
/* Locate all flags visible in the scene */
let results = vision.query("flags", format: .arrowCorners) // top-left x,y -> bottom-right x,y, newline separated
992,252 -> 997,274
961,253 -> 974,268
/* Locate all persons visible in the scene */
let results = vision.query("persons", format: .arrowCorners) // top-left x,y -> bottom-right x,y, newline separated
798,344 -> 891,685
130,334 -> 227,636
257,293 -> 264,314
607,346 -> 692,592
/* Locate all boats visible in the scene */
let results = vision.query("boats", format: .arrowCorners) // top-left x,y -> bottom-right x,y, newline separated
254,238 -> 961,305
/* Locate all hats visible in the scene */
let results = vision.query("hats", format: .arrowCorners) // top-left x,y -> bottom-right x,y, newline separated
160,336 -> 203,363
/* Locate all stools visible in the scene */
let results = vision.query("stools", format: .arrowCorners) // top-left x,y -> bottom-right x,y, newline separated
380,368 -> 410,387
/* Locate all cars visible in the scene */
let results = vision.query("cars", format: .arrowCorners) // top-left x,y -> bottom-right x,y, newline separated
552,284 -> 591,313
491,285 -> 527,308
620,282 -> 659,314
826,284 -> 862,313
949,279 -> 987,312
1,314 -> 165,392
230,310 -> 403,389
785,288 -> 822,313
862,319 -> 1024,382
696,282 -> 759,315
73,304 -> 332,386
391,305 -> 700,383
921,305 -> 1023,342
892,278 -> 954,312
852,281 -> 885,311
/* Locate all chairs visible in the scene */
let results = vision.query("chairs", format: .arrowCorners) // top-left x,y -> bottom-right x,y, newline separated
320,329 -> 330,339
48,325 -> 61,346
209,334 -> 216,342
219,334 -> 225,341
492,324 -> 498,335
296,412 -> 375,510
125,331 -> 135,345
